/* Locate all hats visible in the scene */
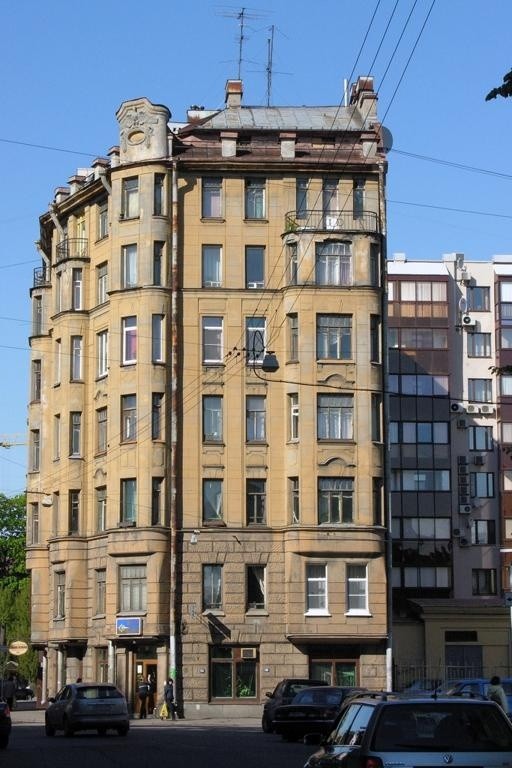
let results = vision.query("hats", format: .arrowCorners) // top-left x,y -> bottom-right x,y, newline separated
490,676 -> 500,685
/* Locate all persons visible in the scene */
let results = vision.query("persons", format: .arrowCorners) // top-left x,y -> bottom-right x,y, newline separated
164,679 -> 177,721
487,676 -> 508,715
76,677 -> 82,683
137,683 -> 151,719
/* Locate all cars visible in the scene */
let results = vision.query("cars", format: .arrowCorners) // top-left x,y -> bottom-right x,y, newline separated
303,690 -> 512,768
45,683 -> 129,738
0,688 -> 35,751
261,678 -> 512,743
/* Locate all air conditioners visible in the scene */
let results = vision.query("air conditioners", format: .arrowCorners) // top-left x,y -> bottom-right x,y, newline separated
458,455 -> 472,514
474,454 -> 486,465
461,316 -> 477,326
449,401 -> 494,415
456,270 -> 472,281
452,528 -> 472,548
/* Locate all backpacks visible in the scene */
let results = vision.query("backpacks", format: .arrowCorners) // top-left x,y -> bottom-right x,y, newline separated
488,688 -> 502,706
